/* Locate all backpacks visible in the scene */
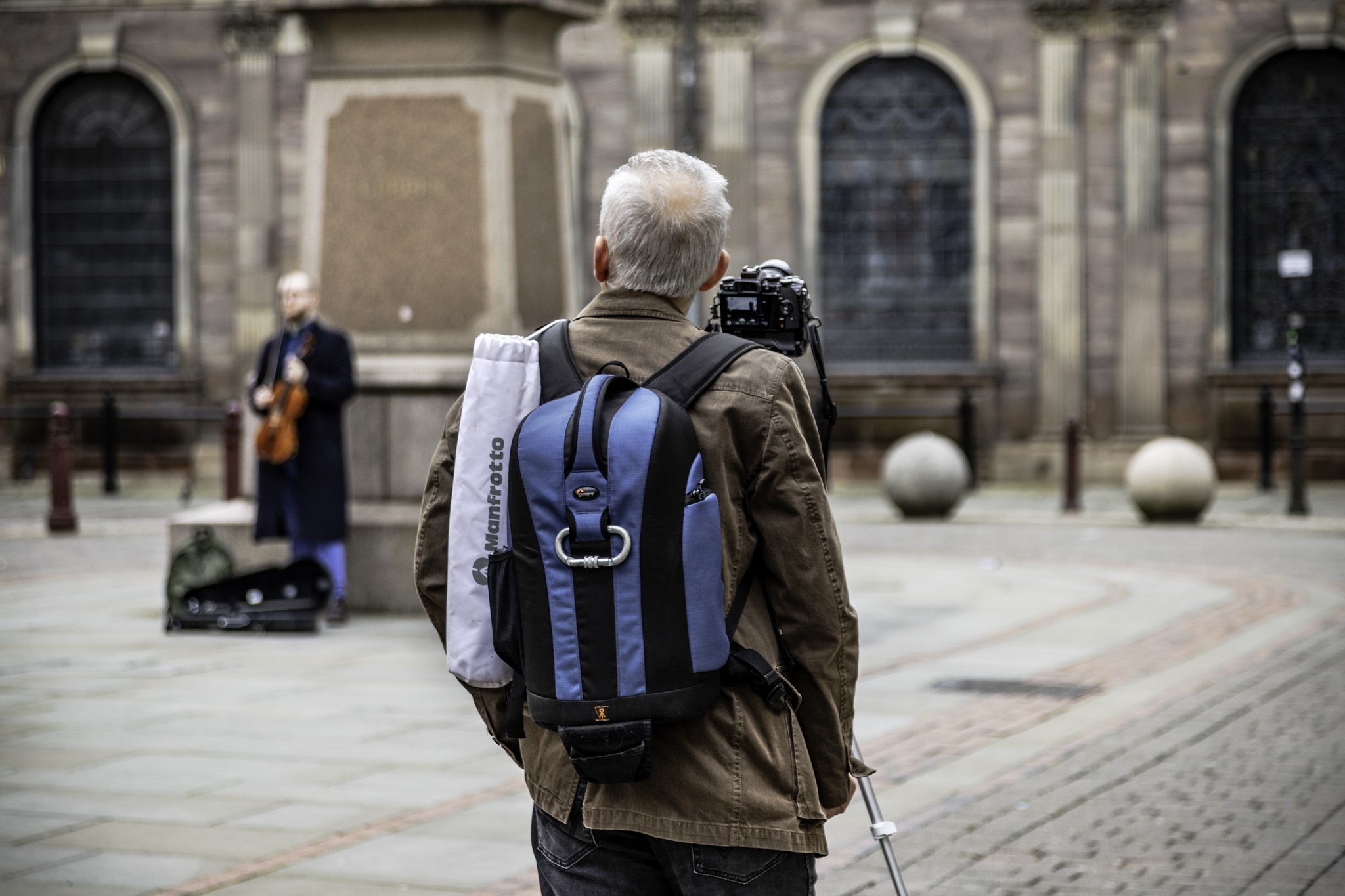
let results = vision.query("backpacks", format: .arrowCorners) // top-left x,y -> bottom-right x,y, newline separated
478,317 -> 759,777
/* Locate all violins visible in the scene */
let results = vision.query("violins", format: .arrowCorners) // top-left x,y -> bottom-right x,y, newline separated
254,329 -> 317,465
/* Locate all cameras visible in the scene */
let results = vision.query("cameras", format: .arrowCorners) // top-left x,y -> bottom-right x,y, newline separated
716,258 -> 812,356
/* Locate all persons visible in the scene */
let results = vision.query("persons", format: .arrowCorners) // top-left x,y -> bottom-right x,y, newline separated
252,268 -> 358,629
413,148 -> 878,896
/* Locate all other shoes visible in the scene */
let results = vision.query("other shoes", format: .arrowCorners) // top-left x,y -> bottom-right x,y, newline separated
327,596 -> 347,623
